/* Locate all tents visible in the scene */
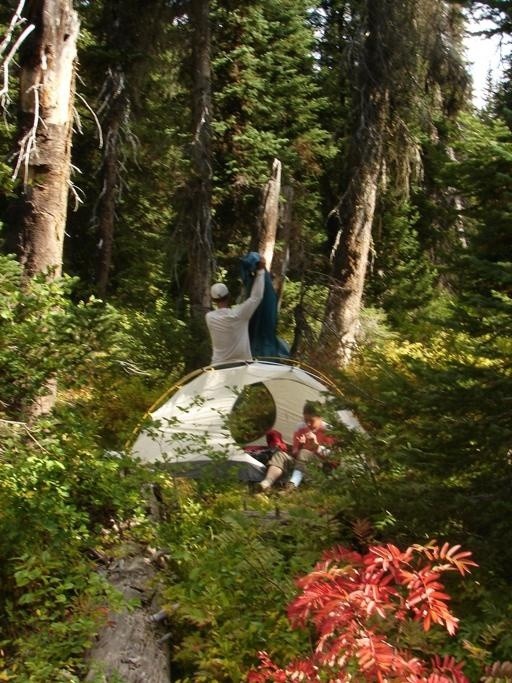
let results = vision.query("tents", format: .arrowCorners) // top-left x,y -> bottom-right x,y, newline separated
117,353 -> 376,485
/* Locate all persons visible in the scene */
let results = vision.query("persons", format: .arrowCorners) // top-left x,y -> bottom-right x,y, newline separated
255,400 -> 342,499
204,256 -> 266,366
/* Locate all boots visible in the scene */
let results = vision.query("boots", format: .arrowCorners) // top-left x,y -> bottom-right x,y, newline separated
253,482 -> 295,502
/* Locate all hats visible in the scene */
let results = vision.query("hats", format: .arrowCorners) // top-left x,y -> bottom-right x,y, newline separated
210,283 -> 229,299
